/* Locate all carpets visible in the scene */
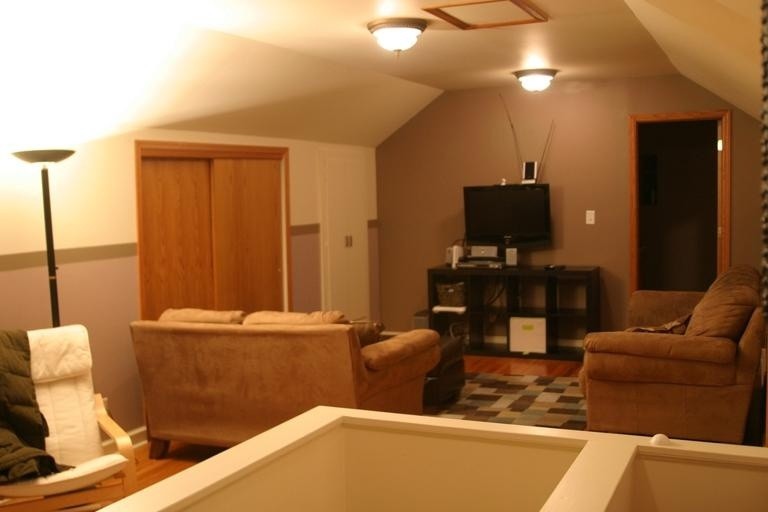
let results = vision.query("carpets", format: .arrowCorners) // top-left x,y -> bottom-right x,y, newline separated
454,366 -> 589,433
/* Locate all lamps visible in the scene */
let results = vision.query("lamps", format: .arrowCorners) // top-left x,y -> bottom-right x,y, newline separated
13,148 -> 77,324
366,16 -> 428,60
513,66 -> 559,91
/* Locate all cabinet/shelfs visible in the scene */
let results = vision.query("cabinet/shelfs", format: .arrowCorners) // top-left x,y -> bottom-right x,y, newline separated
428,262 -> 601,367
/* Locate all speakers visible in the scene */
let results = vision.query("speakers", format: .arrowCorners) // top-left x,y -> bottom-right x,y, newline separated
521,160 -> 537,184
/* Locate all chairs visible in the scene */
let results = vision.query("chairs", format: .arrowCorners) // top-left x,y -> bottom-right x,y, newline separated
0,324 -> 135,512
578,288 -> 764,446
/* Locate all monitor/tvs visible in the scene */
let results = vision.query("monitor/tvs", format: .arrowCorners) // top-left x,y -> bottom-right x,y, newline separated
463,183 -> 553,251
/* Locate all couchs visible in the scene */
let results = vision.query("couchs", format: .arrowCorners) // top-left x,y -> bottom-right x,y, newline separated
123,310 -> 447,461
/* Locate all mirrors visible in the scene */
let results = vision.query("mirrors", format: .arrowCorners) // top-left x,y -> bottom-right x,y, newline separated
628,110 -> 734,292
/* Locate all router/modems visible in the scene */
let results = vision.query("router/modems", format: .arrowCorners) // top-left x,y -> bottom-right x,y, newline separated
452,245 -> 462,269
